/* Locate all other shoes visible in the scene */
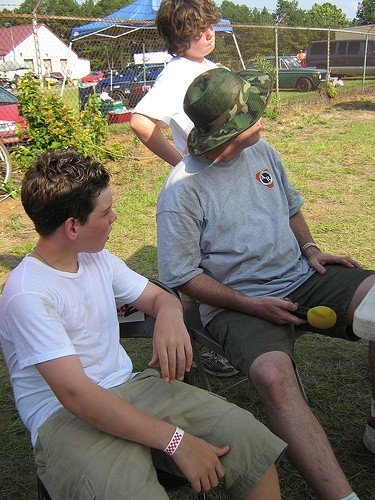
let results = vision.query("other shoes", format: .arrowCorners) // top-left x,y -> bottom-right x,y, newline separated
362,423 -> 375,454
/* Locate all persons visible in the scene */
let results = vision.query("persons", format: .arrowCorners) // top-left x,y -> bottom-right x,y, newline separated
156,67 -> 375,500
297,50 -> 306,69
130,0 -> 241,378
0,149 -> 289,500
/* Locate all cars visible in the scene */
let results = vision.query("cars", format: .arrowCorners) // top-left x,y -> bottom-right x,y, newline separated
0,86 -> 31,151
37,72 -> 68,87
94,62 -> 167,106
82,72 -> 104,83
246,56 -> 327,92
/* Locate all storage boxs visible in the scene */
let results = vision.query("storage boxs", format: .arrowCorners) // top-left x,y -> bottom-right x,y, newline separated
108,111 -> 131,124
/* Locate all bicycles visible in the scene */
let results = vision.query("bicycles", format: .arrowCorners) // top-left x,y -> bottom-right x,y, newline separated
0,142 -> 11,188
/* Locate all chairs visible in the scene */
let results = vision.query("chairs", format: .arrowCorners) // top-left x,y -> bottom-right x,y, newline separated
174,289 -> 308,403
115,279 -> 213,392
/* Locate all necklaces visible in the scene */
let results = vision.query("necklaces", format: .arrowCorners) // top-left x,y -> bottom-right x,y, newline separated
33,247 -> 58,269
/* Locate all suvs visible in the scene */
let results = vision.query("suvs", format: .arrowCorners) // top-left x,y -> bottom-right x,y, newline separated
0,61 -> 33,90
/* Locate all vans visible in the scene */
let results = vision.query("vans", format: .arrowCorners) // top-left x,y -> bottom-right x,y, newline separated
306,38 -> 375,78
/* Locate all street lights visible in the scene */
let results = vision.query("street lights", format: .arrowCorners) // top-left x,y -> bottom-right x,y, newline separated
5,22 -> 16,60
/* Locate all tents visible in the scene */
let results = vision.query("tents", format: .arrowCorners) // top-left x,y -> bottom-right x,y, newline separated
60,0 -> 248,98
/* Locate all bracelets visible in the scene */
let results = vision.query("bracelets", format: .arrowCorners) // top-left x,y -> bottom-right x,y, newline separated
302,242 -> 320,256
164,426 -> 184,456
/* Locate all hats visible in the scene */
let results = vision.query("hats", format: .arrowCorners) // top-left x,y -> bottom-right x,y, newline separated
182,68 -> 274,156
